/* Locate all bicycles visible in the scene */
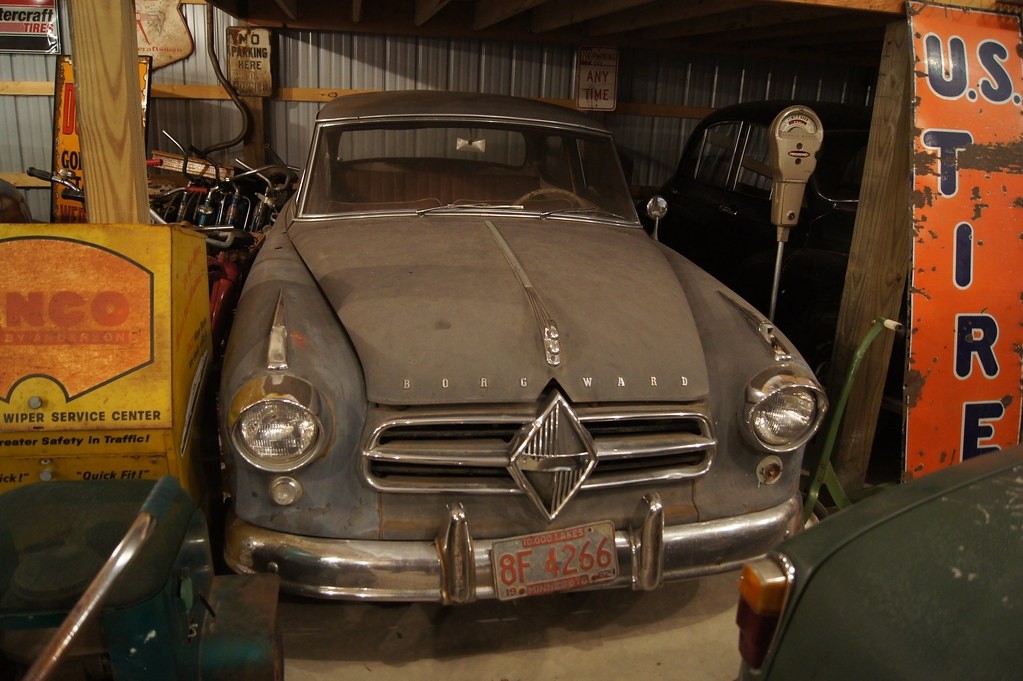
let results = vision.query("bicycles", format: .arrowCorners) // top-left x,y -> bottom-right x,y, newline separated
27,127 -> 302,362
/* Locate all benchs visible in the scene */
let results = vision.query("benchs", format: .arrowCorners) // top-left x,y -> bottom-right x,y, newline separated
334,167 -> 537,206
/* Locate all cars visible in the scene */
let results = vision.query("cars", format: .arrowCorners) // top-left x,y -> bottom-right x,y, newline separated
644,101 -> 903,429
188,85 -> 828,600
731,444 -> 1023,680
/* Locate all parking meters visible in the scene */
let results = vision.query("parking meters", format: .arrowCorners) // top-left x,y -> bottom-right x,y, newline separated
768,104 -> 820,325
